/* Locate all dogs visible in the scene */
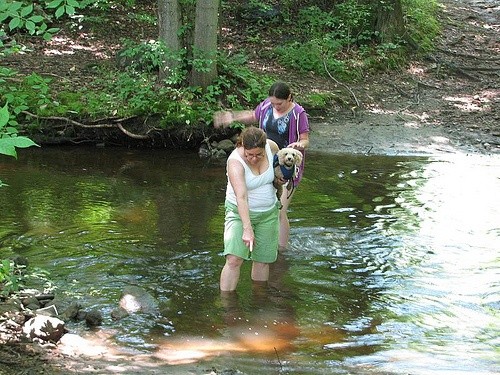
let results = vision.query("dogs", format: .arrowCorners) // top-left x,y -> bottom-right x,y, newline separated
273,147 -> 304,210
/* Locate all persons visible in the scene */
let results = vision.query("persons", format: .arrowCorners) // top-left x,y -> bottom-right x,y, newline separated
217,251 -> 300,356
213,82 -> 309,247
219,126 -> 288,291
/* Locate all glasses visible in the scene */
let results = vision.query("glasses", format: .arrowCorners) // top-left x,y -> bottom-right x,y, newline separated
246,151 -> 265,159
